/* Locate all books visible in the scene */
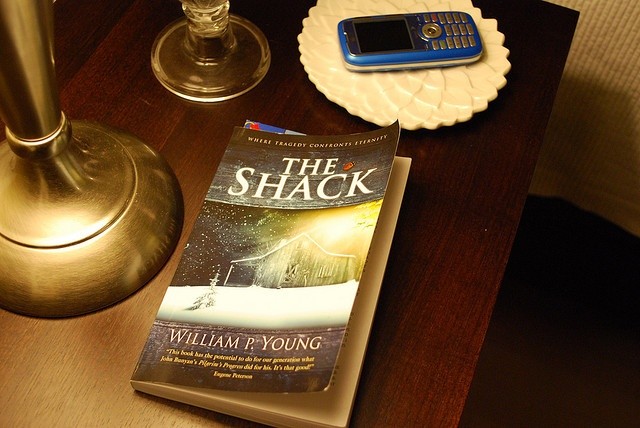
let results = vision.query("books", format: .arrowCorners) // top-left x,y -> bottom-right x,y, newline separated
128,120 -> 414,428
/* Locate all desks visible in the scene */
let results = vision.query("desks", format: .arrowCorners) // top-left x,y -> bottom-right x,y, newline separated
1,0 -> 580,428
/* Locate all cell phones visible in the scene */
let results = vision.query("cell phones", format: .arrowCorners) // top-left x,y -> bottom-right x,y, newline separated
337,11 -> 484,73
